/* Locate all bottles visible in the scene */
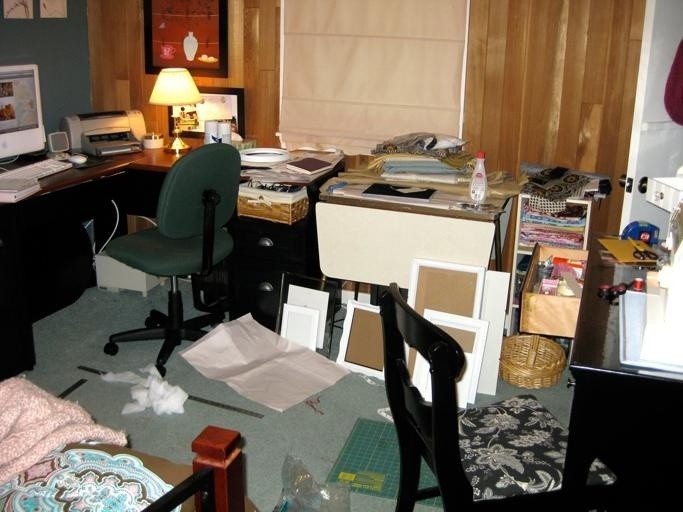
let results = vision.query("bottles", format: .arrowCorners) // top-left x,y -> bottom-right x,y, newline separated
469,152 -> 487,204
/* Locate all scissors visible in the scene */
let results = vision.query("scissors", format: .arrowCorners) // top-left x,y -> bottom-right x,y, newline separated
627,236 -> 659,262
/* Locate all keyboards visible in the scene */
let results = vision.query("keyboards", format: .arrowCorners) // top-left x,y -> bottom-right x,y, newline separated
1,159 -> 73,180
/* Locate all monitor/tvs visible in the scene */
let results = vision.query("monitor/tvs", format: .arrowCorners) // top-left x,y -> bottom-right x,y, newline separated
1,64 -> 46,158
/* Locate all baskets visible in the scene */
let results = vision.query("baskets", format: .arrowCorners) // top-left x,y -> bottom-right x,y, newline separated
499,334 -> 566,390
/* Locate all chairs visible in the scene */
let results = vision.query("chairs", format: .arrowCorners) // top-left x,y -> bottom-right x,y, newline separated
380,281 -> 617,511
95,145 -> 242,376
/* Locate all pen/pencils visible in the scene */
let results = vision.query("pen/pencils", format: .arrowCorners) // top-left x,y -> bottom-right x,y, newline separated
326,182 -> 347,191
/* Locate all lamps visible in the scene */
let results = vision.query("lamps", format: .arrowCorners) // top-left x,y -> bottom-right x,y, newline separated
148,68 -> 204,154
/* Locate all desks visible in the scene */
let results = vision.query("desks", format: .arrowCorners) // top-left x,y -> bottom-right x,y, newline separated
315,167 -> 518,358
516,231 -> 681,512
0,136 -> 347,378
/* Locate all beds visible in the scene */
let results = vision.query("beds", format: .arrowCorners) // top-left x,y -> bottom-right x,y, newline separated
0,375 -> 259,512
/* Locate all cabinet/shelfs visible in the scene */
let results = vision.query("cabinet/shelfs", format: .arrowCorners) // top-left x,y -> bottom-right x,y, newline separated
505,191 -> 592,356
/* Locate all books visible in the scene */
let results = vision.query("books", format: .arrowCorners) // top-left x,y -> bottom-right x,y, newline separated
0,176 -> 42,202
363,182 -> 436,202
616,287 -> 683,378
287,158 -> 331,174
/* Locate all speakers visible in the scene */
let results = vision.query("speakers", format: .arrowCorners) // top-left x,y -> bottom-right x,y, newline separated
47,132 -> 69,160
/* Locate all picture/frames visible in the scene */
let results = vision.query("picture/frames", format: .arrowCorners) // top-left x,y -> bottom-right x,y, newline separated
39,0 -> 67,18
286,283 -> 330,350
3,0 -> 33,19
143,0 -> 230,80
336,298 -> 386,381
405,257 -> 485,383
280,302 -> 320,352
475,270 -> 511,396
425,349 -> 474,407
412,308 -> 489,410
167,85 -> 246,142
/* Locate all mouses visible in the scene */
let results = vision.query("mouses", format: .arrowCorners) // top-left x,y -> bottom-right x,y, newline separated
69,155 -> 87,164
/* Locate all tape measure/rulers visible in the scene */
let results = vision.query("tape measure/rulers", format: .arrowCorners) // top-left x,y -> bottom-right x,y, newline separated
57,378 -> 87,399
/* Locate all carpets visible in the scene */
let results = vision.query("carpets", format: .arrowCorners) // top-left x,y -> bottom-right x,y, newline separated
323,416 -> 444,509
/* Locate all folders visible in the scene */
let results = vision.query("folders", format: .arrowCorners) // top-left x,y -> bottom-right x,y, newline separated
597,238 -> 663,263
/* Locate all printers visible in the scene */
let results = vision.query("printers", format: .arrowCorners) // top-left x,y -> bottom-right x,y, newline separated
62,110 -> 146,156
